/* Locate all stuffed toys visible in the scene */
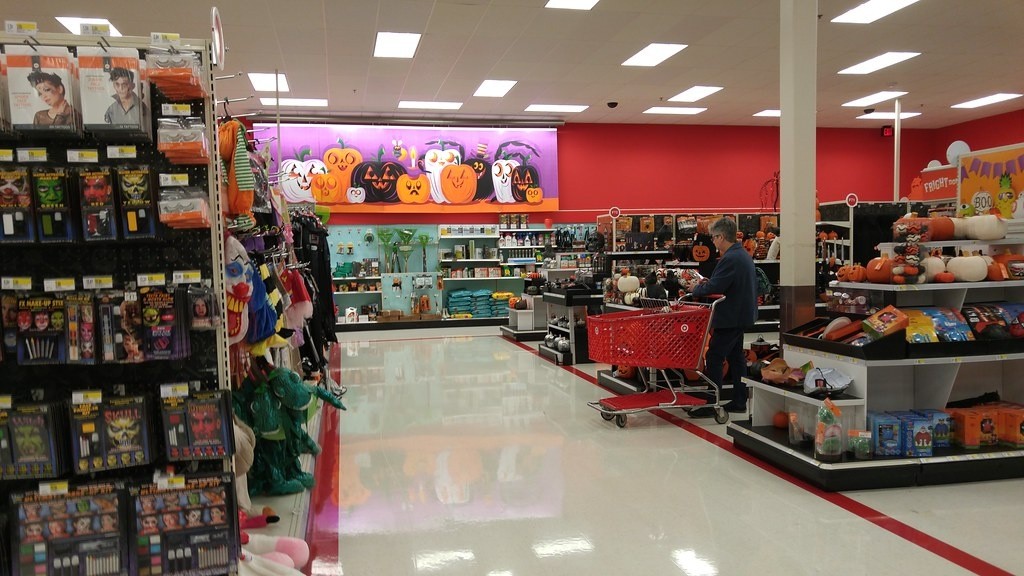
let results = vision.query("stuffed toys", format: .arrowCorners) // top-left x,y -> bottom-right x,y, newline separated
232,365 -> 347,498
232,414 -> 310,576
606,267 -> 681,308
864,203 -> 1023,282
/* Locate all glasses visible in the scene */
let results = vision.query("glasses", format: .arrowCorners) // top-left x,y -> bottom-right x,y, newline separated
711,235 -> 720,241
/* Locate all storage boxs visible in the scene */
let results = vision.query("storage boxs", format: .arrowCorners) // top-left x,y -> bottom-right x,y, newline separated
400,314 -> 420,320
390,310 -> 403,316
381,310 -> 391,316
377,316 -> 399,322
421,314 -> 442,320
862,305 -> 908,340
867,400 -> 1024,457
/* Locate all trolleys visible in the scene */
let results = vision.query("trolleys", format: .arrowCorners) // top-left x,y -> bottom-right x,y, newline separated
586,293 -> 729,428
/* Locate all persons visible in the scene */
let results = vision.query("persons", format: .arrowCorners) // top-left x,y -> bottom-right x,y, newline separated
135,487 -> 226,535
118,332 -> 144,362
19,494 -> 120,544
27,69 -> 147,129
194,299 -> 210,319
686,216 -> 759,419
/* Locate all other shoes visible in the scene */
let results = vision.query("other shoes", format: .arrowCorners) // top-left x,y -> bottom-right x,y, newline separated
687,405 -> 720,418
721,401 -> 748,414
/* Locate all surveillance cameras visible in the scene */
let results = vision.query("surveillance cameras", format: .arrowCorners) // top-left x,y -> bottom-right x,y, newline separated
607,102 -> 618,108
864,109 -> 874,114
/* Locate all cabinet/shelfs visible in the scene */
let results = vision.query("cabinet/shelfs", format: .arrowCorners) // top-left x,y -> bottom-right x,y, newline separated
439,229 -> 560,330
500,268 -> 592,340
332,276 -> 381,323
726,238 -> 1024,489
596,261 -> 780,395
539,250 -> 673,365
